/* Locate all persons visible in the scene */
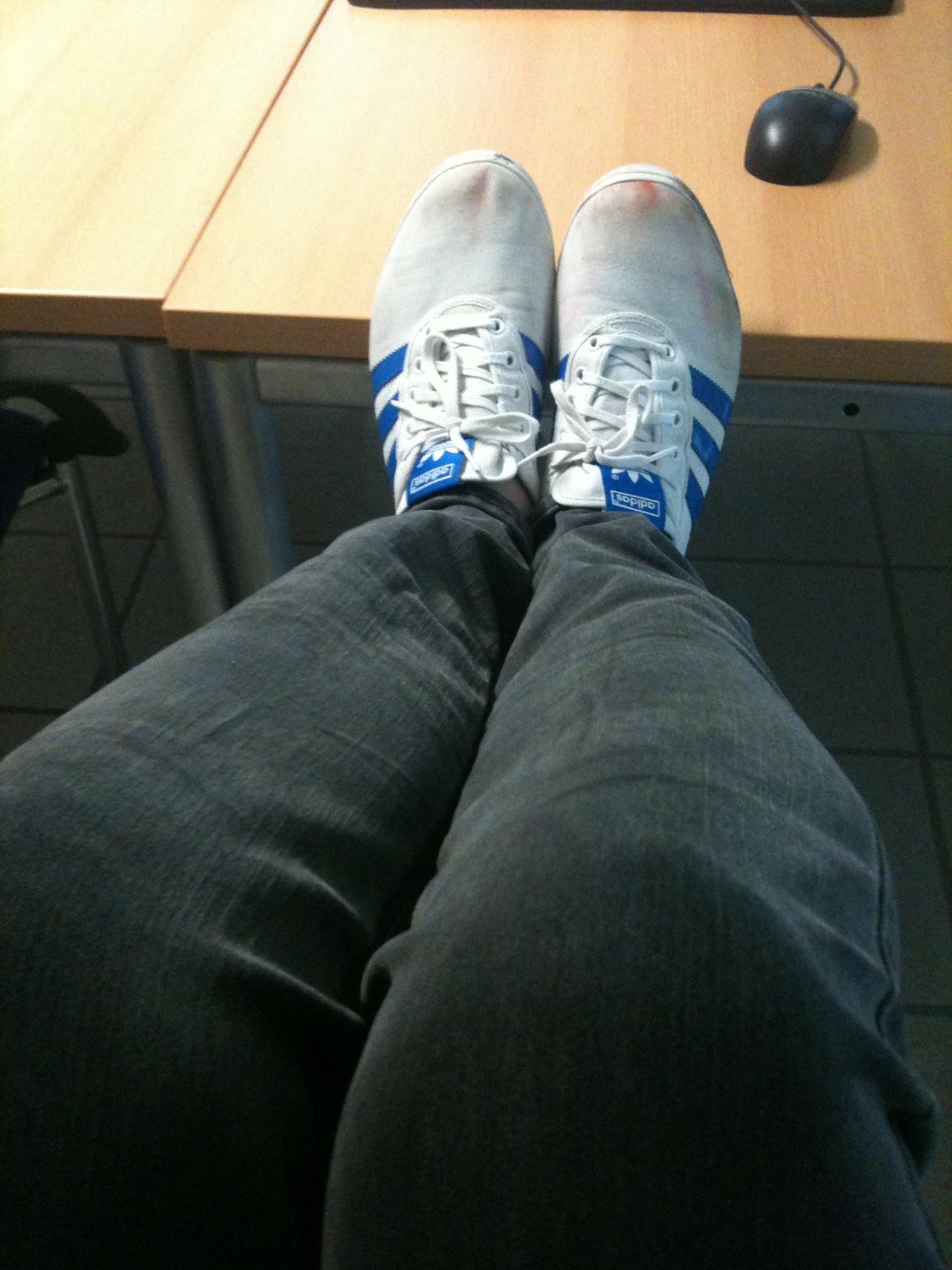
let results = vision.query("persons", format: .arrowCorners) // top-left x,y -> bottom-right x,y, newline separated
0,149 -> 940,1270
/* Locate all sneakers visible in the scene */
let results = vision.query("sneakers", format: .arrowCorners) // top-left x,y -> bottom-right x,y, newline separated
552,164 -> 741,565
366,150 -> 556,519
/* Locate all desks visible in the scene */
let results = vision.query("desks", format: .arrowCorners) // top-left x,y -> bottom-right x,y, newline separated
0,0 -> 335,634
160,0 -> 952,607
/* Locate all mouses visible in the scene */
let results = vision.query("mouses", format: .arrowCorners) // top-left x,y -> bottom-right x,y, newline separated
744,83 -> 860,186
